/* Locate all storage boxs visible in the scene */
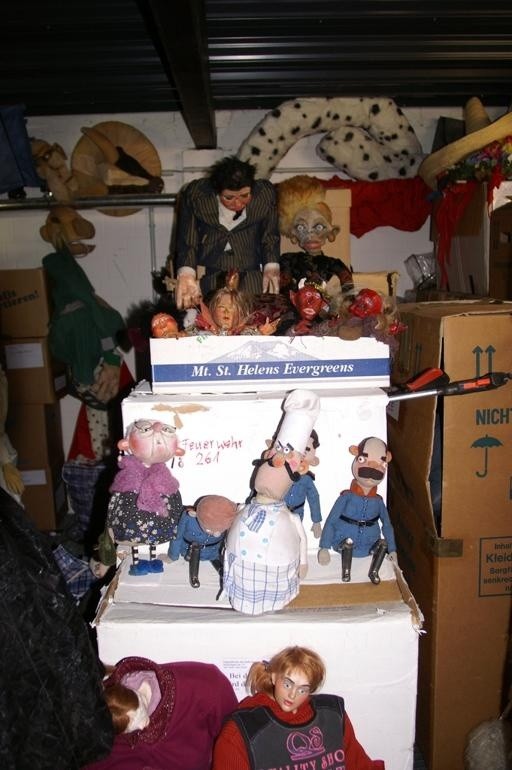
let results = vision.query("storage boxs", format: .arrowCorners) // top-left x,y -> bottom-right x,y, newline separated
280,186 -> 353,267
120,378 -> 390,552
392,537 -> 511,769
385,297 -> 511,541
404,182 -> 512,299
93,559 -> 419,769
149,328 -> 390,394
